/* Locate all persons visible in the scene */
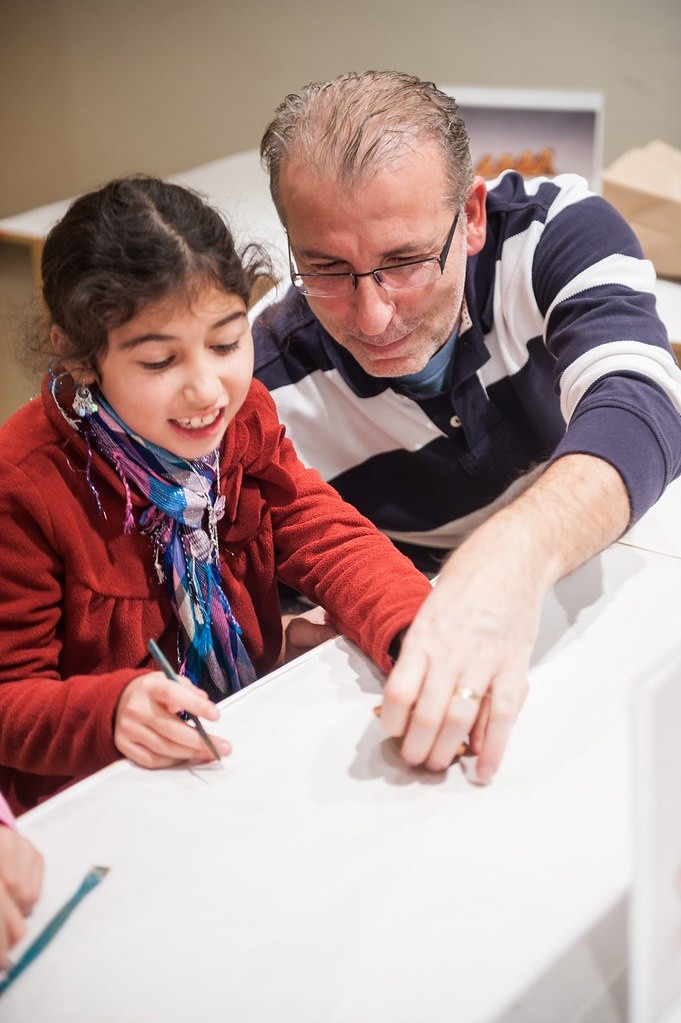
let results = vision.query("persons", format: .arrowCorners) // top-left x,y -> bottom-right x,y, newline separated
0,178 -> 490,819
250,69 -> 681,780
0,793 -> 44,973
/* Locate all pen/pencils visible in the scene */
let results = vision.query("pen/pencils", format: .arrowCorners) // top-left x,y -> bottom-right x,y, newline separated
146,637 -> 223,764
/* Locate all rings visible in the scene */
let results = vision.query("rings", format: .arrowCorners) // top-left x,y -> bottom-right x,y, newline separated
456,684 -> 493,703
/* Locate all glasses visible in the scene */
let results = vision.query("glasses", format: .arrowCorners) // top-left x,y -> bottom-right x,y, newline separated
286,200 -> 461,300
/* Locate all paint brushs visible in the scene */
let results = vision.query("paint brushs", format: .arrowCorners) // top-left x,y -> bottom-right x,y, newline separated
1,865 -> 109,991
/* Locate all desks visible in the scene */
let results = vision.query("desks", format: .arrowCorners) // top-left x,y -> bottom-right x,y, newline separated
0,476 -> 678,1022
0,148 -> 681,373
